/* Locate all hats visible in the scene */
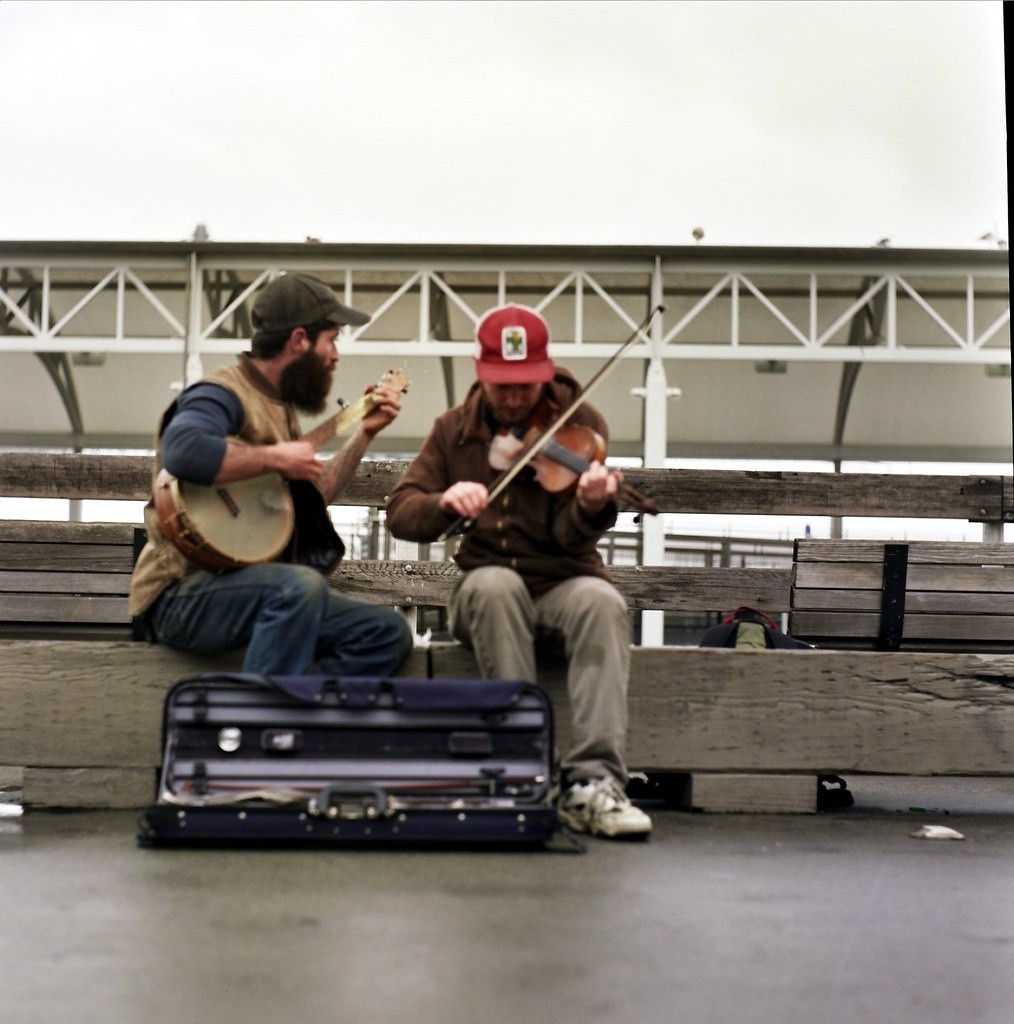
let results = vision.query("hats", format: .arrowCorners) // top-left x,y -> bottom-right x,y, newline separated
251,272 -> 371,333
472,302 -> 555,383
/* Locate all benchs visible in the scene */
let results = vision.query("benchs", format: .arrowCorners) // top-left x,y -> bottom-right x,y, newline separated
0,451 -> 1014,815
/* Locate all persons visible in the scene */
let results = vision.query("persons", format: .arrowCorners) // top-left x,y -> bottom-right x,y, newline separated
385,303 -> 653,836
127,274 -> 414,676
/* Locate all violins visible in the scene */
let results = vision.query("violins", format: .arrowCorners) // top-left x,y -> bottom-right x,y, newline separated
488,398 -> 661,524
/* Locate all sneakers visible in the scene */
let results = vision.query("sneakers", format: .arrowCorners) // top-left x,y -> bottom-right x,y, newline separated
560,774 -> 653,839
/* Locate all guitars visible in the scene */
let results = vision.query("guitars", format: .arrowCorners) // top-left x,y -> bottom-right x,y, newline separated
153,367 -> 410,571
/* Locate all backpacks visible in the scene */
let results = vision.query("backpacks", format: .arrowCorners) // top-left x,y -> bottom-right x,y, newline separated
700,605 -> 816,650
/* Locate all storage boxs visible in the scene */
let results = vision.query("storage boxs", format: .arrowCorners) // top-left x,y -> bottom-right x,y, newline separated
137,673 -> 561,851
